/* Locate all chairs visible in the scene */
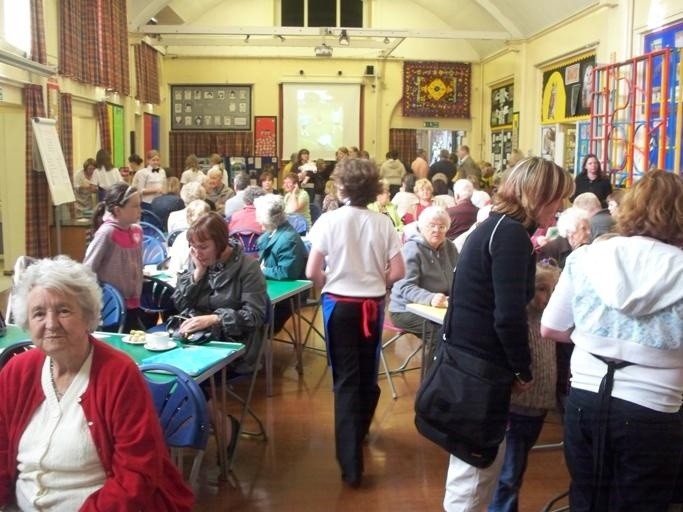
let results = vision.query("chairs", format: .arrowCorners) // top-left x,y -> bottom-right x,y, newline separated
0,193 -> 454,512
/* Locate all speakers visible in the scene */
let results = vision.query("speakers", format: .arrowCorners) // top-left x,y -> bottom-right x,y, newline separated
367,65 -> 374,74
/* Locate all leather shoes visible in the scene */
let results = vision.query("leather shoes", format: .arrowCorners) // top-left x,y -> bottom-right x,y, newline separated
218,414 -> 240,466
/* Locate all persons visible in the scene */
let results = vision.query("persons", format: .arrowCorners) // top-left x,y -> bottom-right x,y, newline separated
73,143 -> 325,465
0,252 -> 196,512
307,145 -> 682,511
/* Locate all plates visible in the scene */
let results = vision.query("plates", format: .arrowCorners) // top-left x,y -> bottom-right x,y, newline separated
119,334 -> 148,345
144,340 -> 176,351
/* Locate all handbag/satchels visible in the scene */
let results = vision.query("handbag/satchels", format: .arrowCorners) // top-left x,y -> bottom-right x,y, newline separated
415,333 -> 512,468
165,307 -> 222,345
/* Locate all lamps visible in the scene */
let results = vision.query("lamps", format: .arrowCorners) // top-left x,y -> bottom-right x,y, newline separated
237,28 -> 393,48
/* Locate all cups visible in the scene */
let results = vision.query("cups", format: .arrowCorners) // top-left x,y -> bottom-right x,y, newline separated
151,331 -> 169,348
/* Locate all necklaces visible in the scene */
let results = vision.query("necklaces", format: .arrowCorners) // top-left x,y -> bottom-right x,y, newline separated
50,359 -> 66,398
